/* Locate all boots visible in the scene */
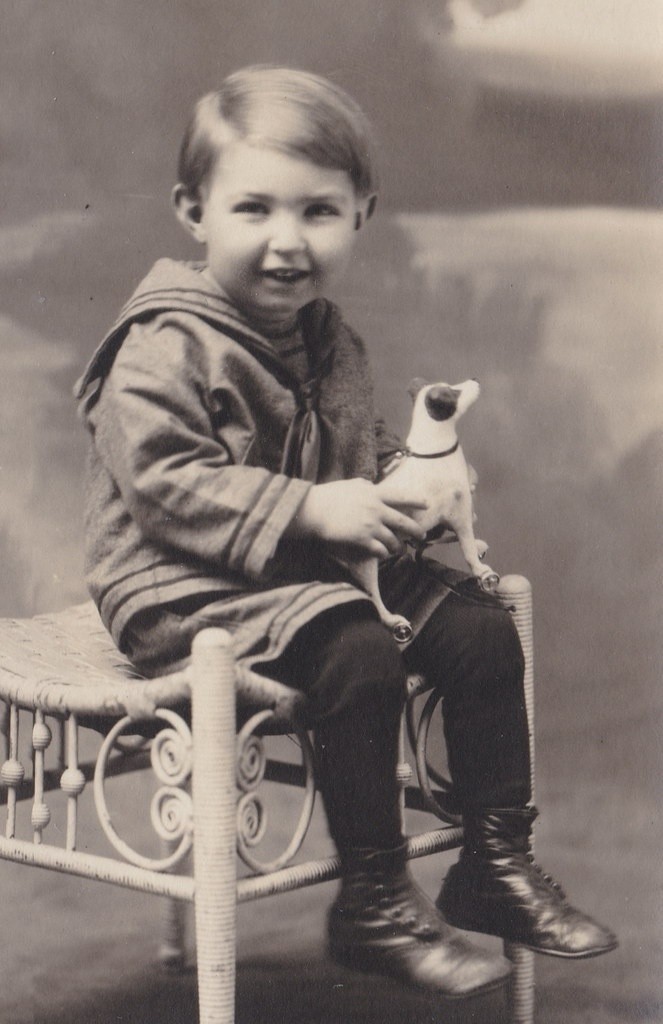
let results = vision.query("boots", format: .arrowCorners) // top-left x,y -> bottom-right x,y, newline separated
435,809 -> 620,959
328,836 -> 515,1003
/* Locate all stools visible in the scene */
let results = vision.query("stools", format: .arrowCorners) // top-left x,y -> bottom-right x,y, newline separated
0,573 -> 535,1024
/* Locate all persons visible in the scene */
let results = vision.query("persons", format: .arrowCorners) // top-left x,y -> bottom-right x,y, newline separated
74,67 -> 620,1000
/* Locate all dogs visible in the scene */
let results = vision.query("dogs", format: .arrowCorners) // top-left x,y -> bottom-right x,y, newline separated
346,376 -> 501,644
